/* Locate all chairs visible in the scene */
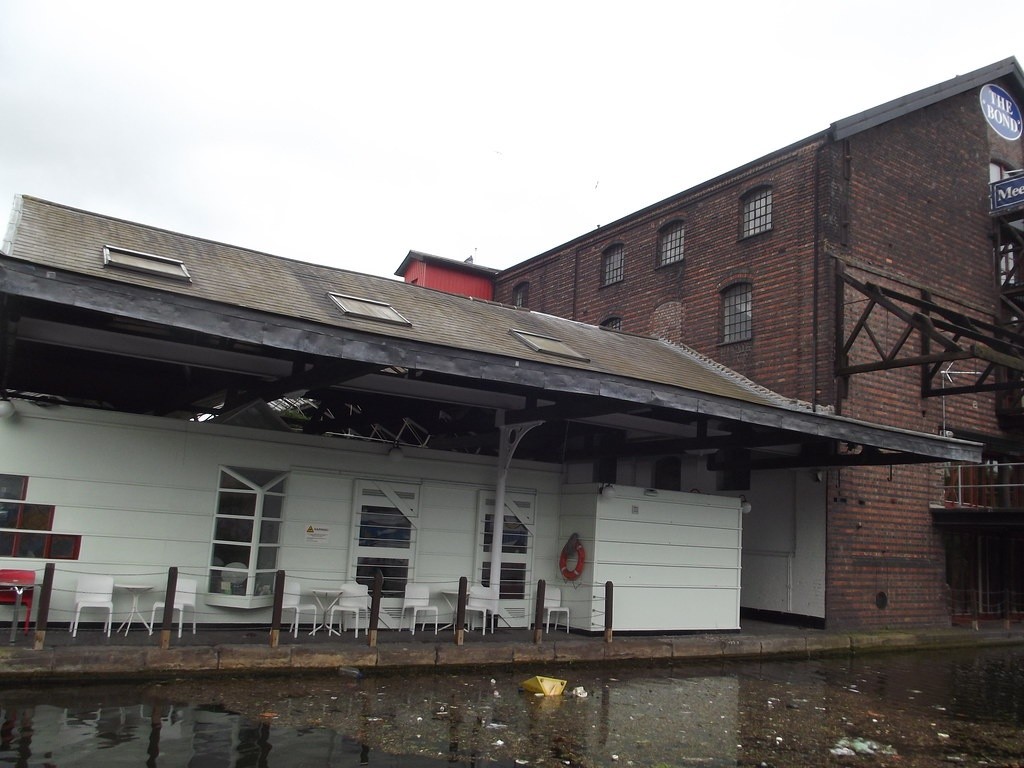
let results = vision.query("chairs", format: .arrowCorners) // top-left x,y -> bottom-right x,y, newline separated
149,579 -> 197,638
544,588 -> 569,634
0,568 -> 35,637
69,575 -> 114,637
399,583 -> 438,635
465,587 -> 497,635
270,582 -> 317,638
329,584 -> 368,638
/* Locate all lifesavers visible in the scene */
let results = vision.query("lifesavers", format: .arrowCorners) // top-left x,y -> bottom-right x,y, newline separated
559,542 -> 585,580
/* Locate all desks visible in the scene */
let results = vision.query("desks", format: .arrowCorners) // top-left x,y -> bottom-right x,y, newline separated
114,584 -> 154,637
0,583 -> 42,643
308,590 -> 344,636
438,591 -> 470,633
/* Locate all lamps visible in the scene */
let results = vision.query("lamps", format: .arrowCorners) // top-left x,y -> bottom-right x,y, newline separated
389,440 -> 404,462
599,479 -> 616,499
0,390 -> 15,419
739,494 -> 751,514
